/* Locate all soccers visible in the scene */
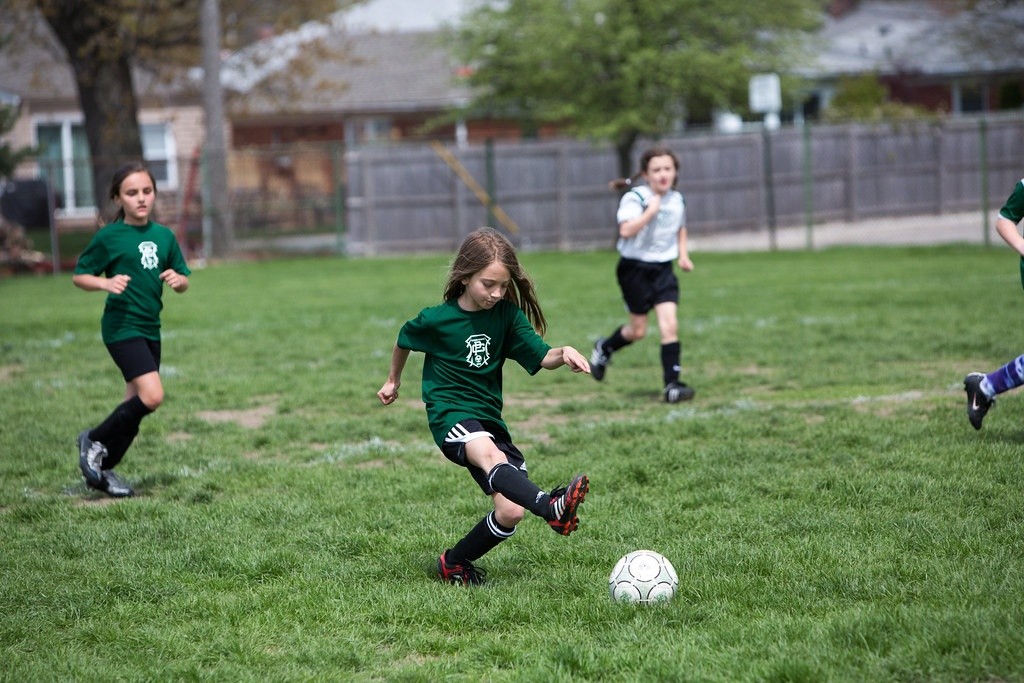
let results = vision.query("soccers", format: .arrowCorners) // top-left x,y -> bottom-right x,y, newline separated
608,548 -> 680,613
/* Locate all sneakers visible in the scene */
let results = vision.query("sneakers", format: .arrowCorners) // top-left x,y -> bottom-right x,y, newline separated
590,339 -> 611,382
664,383 -> 693,403
77,432 -> 109,483
437,549 -> 486,585
86,470 -> 132,495
545,475 -> 589,538
964,372 -> 995,432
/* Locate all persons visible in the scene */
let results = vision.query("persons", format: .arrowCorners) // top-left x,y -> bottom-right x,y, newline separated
589,148 -> 696,404
73,163 -> 192,497
376,227 -> 591,585
963,177 -> 1024,432
